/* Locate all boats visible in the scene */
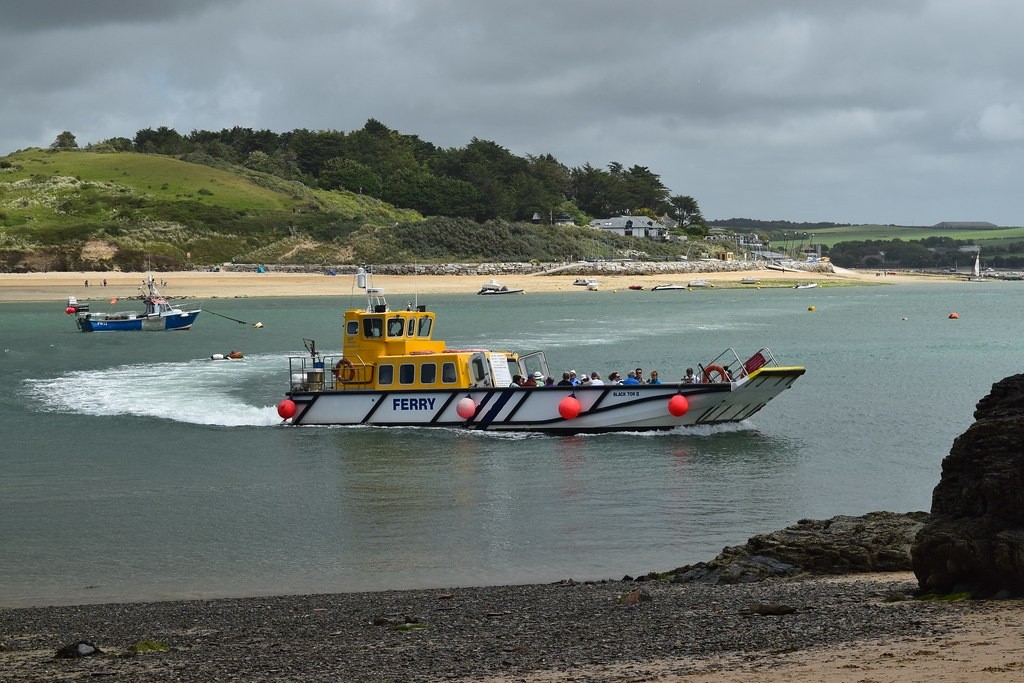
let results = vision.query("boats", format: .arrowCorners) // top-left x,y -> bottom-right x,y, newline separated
740,277 -> 758,284
650,283 -> 686,290
793,283 -> 818,289
477,279 -> 525,296
571,277 -> 589,286
588,279 -> 599,291
62,250 -> 207,331
277,261 -> 809,434
687,281 -> 715,288
629,285 -> 644,291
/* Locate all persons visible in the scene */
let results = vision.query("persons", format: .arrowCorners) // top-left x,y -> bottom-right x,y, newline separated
623,370 -> 639,384
683,368 -> 700,383
85,280 -> 88,287
509,372 -> 555,387
649,371 -> 661,384
104,279 -> 107,286
727,370 -> 736,382
557,370 -> 604,386
635,368 -> 646,384
608,372 -> 621,385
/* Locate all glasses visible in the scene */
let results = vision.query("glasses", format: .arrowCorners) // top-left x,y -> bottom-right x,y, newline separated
636,372 -> 641,374
615,376 -> 620,378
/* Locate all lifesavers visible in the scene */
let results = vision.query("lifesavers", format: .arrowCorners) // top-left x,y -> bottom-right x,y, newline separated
466,349 -> 489,352
442,349 -> 465,353
335,358 -> 355,384
410,350 -> 436,355
702,366 -> 727,384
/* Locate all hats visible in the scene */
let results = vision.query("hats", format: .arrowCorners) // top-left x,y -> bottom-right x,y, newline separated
534,371 -> 544,380
569,370 -> 576,375
